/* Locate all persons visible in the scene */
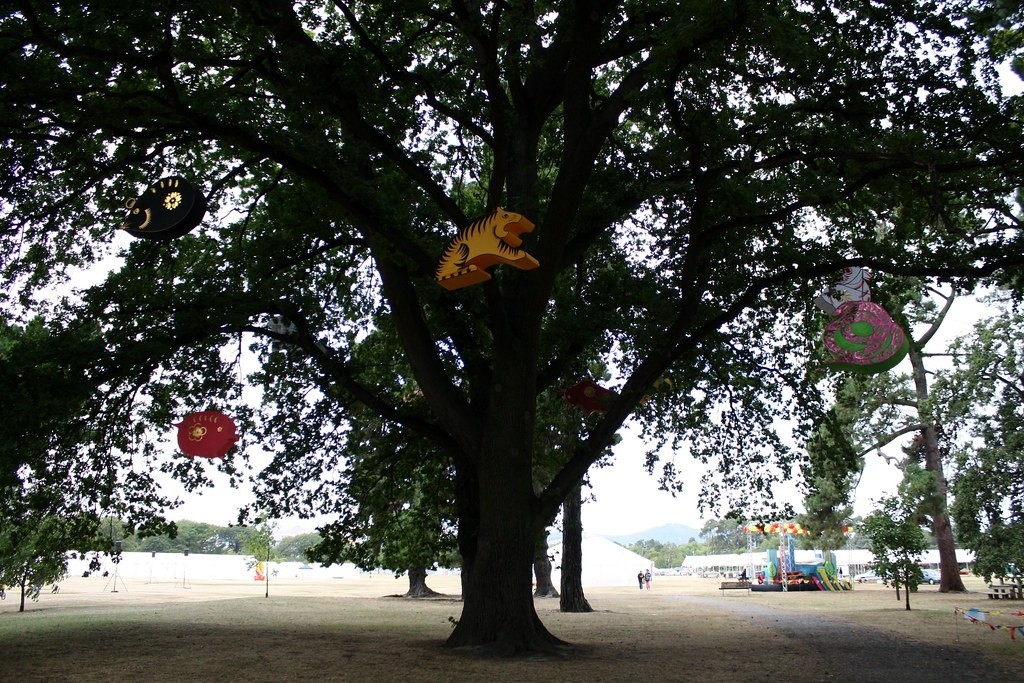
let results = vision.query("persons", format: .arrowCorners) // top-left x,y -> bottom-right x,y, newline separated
739,569 -> 746,585
638,569 -> 651,590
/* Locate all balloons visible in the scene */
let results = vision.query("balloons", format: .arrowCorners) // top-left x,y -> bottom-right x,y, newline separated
744,523 -> 853,534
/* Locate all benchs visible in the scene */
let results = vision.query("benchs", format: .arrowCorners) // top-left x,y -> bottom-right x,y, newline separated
719,582 -> 749,596
983,591 -> 1024,595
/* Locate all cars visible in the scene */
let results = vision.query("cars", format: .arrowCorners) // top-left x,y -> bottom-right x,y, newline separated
853,569 -> 892,583
919,568 -> 942,584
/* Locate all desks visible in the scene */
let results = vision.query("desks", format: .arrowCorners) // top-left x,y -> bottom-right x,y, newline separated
988,583 -> 1024,599
